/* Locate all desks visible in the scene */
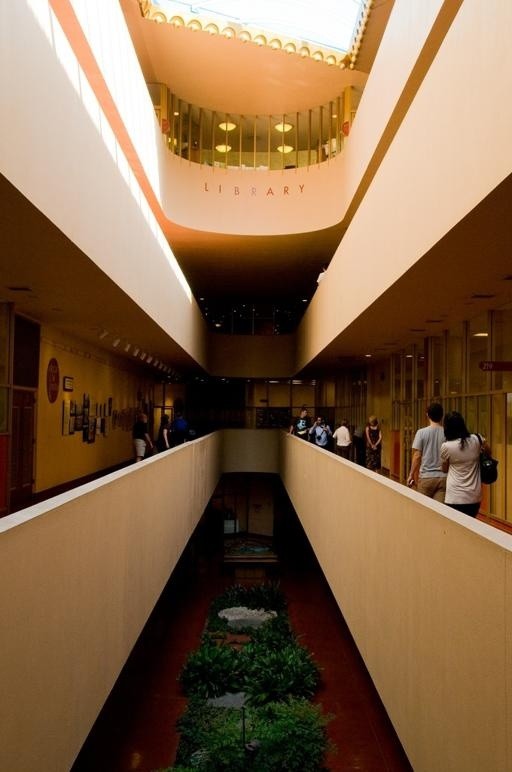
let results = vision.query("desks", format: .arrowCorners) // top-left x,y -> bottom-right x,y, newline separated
223,549 -> 277,590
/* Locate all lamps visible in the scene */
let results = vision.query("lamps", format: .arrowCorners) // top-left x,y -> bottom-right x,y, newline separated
218,113 -> 294,156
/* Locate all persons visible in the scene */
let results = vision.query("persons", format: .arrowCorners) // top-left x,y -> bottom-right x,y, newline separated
158,412 -> 197,451
332,419 -> 353,459
132,412 -> 153,462
308,416 -> 333,450
365,415 -> 382,472
439,411 -> 492,518
406,403 -> 447,505
286,407 -> 312,442
353,423 -> 367,466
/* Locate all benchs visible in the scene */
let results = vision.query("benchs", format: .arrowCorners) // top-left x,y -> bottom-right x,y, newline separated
269,571 -> 296,600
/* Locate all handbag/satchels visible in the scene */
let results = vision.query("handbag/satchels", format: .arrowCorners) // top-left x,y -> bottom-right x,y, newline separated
475,433 -> 498,484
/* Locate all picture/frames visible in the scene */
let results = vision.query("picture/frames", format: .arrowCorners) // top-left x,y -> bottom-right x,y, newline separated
60,391 -> 117,447
